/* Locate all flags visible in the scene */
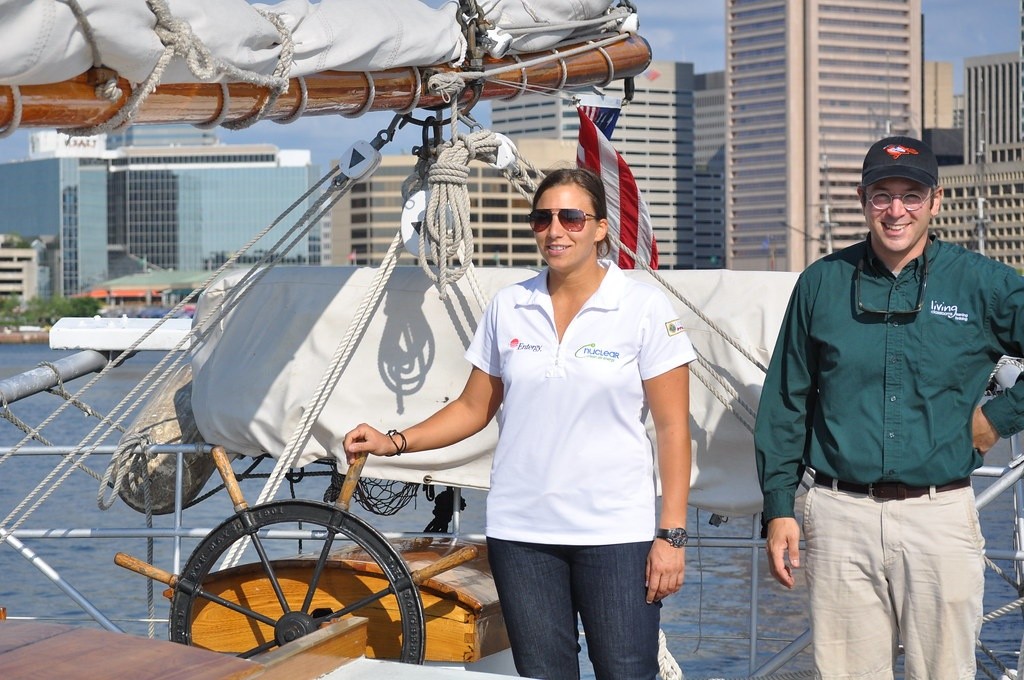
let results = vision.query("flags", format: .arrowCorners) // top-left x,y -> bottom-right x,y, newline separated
570,95 -> 659,270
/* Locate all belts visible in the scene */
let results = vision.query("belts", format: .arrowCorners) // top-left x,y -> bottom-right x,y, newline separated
813,471 -> 970,500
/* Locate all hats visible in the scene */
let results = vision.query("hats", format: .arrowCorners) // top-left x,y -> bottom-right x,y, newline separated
862,137 -> 938,192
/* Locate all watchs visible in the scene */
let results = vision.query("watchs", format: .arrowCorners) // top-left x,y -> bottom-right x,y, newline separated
656,527 -> 689,548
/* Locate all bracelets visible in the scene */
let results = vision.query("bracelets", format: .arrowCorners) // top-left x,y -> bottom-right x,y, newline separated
384,429 -> 408,457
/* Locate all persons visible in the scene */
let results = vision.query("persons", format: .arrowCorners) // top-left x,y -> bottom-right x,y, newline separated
341,168 -> 696,680
752,136 -> 1023,680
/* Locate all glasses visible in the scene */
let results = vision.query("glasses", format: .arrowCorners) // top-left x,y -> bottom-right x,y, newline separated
862,186 -> 940,211
528,208 -> 595,232
